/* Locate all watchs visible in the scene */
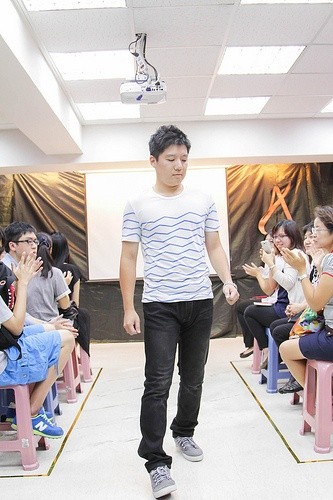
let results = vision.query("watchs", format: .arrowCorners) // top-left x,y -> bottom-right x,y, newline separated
269,265 -> 276,270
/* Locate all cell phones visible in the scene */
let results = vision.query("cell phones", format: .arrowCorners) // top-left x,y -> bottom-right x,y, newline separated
261,241 -> 272,254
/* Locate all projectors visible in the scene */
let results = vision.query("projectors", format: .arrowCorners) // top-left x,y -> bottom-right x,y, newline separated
120,82 -> 167,105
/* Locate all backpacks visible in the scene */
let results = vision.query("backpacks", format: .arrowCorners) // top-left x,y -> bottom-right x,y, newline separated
0,261 -> 23,360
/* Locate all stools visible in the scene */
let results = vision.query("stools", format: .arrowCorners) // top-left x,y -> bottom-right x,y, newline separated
0,340 -> 93,471
297,359 -> 333,454
259,328 -> 291,393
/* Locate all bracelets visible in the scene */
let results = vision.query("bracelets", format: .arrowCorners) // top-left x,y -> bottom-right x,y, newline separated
298,273 -> 308,281
222,282 -> 237,289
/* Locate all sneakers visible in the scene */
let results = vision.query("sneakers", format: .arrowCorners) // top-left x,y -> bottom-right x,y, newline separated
150,465 -> 177,499
6,401 -> 53,422
11,415 -> 64,438
175,437 -> 203,461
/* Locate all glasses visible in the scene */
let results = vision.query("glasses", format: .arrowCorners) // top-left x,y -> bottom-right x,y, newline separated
312,228 -> 331,235
13,239 -> 40,246
273,234 -> 289,240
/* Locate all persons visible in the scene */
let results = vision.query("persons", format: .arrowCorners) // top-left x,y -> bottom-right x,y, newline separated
0,221 -> 92,438
120,125 -> 240,498
234,205 -> 333,394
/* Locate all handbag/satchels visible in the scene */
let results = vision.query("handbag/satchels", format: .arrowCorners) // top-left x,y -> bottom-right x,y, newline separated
288,306 -> 325,339
58,300 -> 78,319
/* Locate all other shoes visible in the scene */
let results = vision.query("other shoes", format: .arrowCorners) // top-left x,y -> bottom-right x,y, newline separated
240,349 -> 253,358
279,376 -> 303,393
